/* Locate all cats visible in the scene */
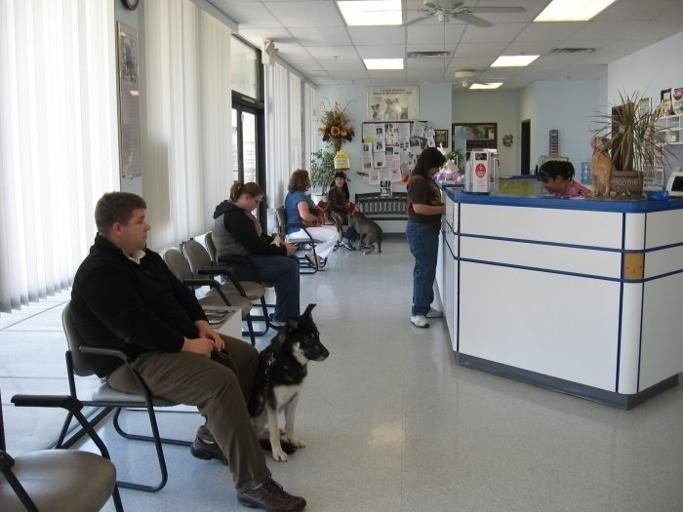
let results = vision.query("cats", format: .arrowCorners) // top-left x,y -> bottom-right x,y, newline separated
370,103 -> 383,121
400,105 -> 409,120
300,199 -> 329,227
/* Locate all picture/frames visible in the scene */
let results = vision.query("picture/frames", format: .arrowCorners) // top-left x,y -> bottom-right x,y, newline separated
634,94 -> 654,188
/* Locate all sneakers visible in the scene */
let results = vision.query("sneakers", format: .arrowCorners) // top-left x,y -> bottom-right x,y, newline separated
426,307 -> 443,318
305,251 -> 327,271
410,314 -> 429,327
341,241 -> 356,252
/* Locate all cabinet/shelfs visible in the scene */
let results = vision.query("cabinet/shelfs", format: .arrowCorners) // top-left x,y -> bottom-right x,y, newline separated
653,113 -> 682,188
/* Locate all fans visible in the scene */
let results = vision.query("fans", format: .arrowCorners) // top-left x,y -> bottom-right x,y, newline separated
395,0 -> 527,29
444,70 -> 489,91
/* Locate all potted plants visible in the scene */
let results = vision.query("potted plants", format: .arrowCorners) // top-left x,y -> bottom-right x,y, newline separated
582,82 -> 681,198
308,142 -> 337,205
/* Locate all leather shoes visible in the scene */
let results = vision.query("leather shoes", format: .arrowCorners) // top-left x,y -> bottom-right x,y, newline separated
237,478 -> 307,512
190,435 -> 229,466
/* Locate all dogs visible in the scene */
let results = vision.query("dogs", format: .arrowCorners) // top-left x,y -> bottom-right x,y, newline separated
590,135 -> 632,198
261,232 -> 285,247
248,302 -> 330,461
339,200 -> 383,258
383,97 -> 399,120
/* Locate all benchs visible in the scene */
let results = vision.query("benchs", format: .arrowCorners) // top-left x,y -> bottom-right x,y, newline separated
354,191 -> 409,223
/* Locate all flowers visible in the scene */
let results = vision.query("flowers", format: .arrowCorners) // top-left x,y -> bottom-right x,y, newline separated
313,95 -> 358,150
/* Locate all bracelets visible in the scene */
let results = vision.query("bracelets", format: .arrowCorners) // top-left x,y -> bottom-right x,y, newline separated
441,206 -> 442,214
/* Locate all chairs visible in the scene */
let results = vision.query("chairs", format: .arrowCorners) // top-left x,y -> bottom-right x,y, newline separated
1,390 -> 125,512
162,231 -> 276,350
55,299 -> 198,494
274,193 -> 345,276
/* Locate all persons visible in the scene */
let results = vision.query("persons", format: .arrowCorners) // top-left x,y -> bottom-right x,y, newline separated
405,147 -> 446,328
211,180 -> 300,327
327,171 -> 355,251
70,191 -> 307,512
284,169 -> 338,271
537,160 -> 592,198
387,124 -> 393,132
